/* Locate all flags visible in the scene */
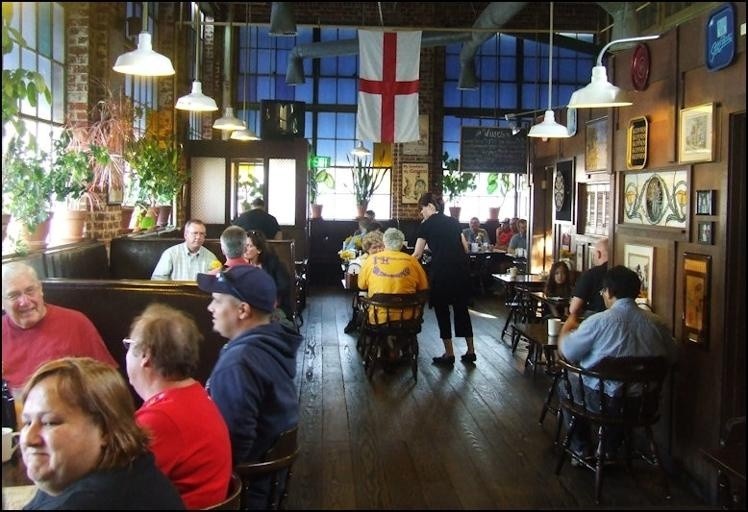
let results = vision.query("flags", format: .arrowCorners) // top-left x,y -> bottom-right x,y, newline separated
352,26 -> 424,146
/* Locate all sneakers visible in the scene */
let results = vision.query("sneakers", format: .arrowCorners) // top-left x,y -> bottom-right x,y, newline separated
432,354 -> 455,364
460,352 -> 477,361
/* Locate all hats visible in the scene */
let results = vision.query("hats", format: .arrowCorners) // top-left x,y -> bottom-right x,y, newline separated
502,218 -> 513,226
196,264 -> 275,313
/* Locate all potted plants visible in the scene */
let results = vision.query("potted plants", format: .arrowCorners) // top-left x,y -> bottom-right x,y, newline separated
438,151 -> 477,222
0,1 -> 193,253
306,163 -> 337,222
344,148 -> 389,219
483,171 -> 514,222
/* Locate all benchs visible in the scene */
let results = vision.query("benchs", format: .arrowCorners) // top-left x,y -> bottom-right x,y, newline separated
204,223 -> 308,304
110,237 -> 295,332
5,280 -> 230,410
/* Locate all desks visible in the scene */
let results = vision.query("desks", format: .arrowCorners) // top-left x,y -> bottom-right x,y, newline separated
465,247 -> 507,299
491,274 -> 570,353
511,324 -> 578,439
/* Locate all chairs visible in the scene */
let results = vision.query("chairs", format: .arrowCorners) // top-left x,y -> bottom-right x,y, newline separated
552,353 -> 678,507
228,424 -> 303,512
357,289 -> 431,385
201,473 -> 242,512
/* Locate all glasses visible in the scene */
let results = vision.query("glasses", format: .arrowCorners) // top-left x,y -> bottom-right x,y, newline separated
216,270 -> 245,301
122,335 -> 144,355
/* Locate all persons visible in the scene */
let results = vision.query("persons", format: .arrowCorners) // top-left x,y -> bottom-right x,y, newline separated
509,218 -> 520,234
350,216 -> 369,238
357,227 -> 431,373
557,264 -> 682,468
348,229 -> 386,315
462,216 -> 491,294
492,217 -> 515,299
14,355 -> 188,510
541,260 -> 571,374
208,225 -> 278,311
194,264 -> 305,511
507,219 -> 527,251
410,192 -> 479,364
2,260 -> 120,403
230,197 -> 280,240
565,237 -> 610,314
364,209 -> 378,224
365,222 -> 385,234
150,218 -> 222,283
117,299 -> 232,511
241,231 -> 294,328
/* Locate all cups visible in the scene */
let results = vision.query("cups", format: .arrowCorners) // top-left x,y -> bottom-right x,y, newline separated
12,383 -> 24,427
506,267 -> 517,277
2,427 -> 21,462
547,318 -> 563,337
507,248 -> 524,256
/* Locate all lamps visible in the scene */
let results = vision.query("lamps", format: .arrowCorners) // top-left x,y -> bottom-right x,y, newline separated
564,30 -> 667,113
504,103 -> 569,137
227,63 -> 263,143
166,0 -> 222,113
208,0 -> 246,134
525,0 -> 575,140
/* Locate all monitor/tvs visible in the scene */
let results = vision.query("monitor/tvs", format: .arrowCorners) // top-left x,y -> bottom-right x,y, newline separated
260,99 -> 305,139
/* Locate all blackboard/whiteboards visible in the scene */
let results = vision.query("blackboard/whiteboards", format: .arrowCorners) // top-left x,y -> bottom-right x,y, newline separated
459,125 -> 529,174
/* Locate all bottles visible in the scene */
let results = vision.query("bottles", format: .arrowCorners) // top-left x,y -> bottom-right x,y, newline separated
2,379 -> 17,432
469,233 -> 495,252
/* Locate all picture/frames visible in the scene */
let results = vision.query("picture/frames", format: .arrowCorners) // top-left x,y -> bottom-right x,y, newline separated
692,188 -> 718,217
695,219 -> 716,247
577,110 -> 613,179
615,239 -> 655,308
574,179 -> 614,241
614,165 -> 693,241
398,159 -> 430,207
673,250 -> 715,345
671,99 -> 720,165
552,158 -> 576,227
